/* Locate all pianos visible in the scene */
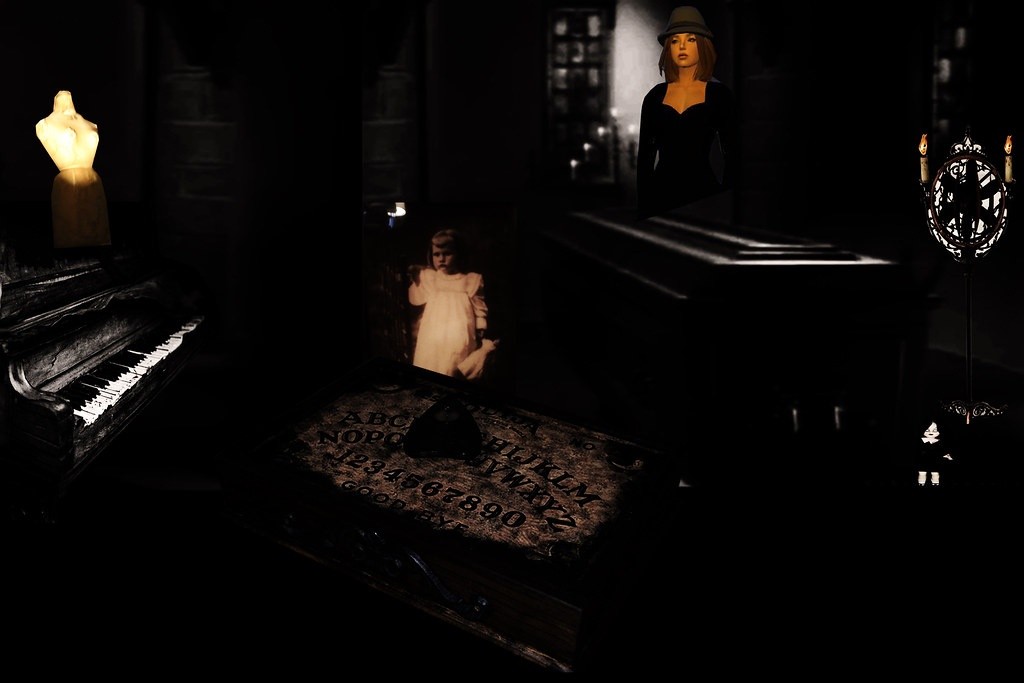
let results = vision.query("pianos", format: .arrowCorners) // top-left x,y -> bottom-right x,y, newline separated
0,257 -> 205,478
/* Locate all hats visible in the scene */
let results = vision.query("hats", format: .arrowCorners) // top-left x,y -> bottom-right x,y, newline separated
658,6 -> 713,46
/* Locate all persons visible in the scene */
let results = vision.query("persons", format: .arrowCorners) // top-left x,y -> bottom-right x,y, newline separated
408,228 -> 500,380
636,6 -> 743,219
34,89 -> 111,248
